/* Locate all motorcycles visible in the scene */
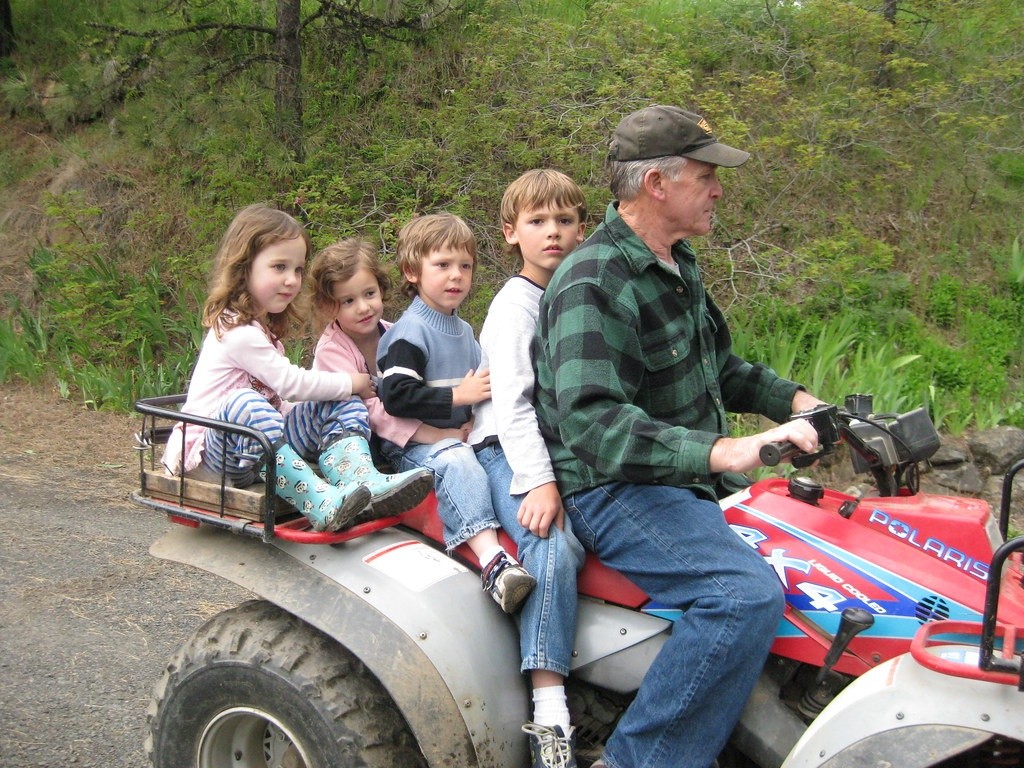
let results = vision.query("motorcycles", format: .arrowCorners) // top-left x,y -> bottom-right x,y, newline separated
125,390 -> 1024,768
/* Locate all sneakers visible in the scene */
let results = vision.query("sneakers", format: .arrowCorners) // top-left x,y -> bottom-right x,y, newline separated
528,722 -> 579,768
480,550 -> 538,615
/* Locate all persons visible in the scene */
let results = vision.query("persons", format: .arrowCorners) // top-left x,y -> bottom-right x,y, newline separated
161,202 -> 435,532
529,104 -> 836,768
466,168 -> 588,768
376,213 -> 537,614
308,238 -> 477,450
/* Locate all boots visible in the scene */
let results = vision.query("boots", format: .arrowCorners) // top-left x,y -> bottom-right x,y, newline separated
318,431 -> 434,525
251,438 -> 372,532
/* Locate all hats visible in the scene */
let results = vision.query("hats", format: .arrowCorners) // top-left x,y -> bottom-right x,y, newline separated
605,106 -> 751,168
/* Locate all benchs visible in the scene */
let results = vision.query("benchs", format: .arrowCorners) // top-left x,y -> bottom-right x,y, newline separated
133,394 -> 324,522
